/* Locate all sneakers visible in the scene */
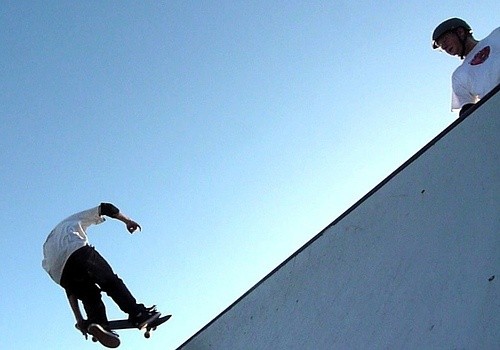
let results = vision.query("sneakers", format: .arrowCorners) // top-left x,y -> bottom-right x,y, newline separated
88,323 -> 120,348
134,310 -> 161,330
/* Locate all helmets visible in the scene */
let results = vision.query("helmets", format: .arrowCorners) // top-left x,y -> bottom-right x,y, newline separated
432,18 -> 472,49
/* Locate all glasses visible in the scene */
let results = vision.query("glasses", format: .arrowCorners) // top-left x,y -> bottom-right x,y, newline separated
436,36 -> 457,47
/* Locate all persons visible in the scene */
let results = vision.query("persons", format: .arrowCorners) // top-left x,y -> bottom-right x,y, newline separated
432,18 -> 500,117
42,203 -> 161,348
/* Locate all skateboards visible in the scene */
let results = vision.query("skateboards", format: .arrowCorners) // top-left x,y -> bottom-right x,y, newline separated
75,314 -> 172,342
